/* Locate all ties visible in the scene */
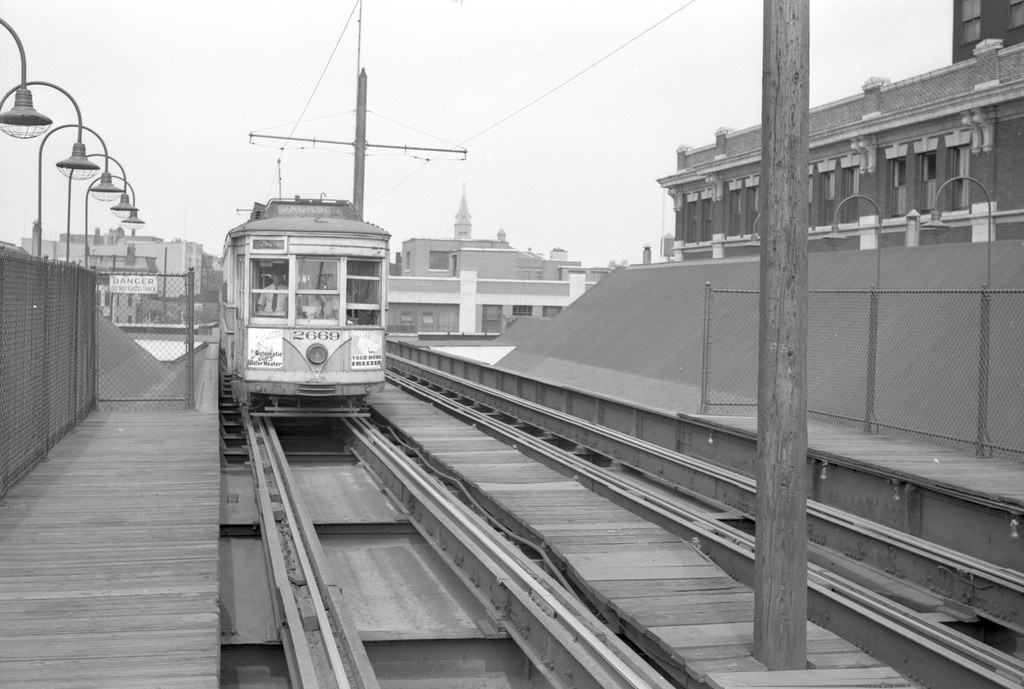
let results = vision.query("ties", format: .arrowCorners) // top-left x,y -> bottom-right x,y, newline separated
272,287 -> 278,311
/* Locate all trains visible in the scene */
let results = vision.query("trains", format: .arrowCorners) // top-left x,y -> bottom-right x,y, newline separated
218,1 -> 393,416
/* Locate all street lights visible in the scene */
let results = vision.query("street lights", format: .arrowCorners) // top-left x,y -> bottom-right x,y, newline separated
38,125 -> 125,267
84,173 -> 146,267
66,153 -> 138,263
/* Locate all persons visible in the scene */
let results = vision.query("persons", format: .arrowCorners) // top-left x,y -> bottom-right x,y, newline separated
256,267 -> 289,320
300,282 -> 335,320
